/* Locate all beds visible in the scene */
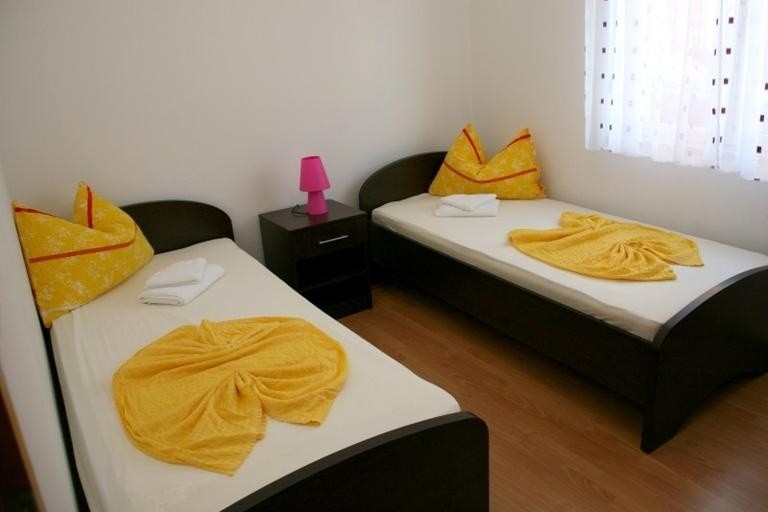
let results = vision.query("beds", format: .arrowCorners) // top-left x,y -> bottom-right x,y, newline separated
358,151 -> 768,457
45,199 -> 492,512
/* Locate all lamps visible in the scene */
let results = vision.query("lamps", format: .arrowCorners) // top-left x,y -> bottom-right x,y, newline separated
300,155 -> 330,215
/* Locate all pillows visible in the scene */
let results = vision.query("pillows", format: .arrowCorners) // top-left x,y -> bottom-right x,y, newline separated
425,121 -> 547,200
10,181 -> 159,332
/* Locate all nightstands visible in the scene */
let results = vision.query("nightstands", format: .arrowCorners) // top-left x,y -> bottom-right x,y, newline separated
259,199 -> 374,321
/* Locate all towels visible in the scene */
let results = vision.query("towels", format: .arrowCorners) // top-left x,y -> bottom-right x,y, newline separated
508,211 -> 704,282
441,193 -> 497,210
110,315 -> 348,477
139,262 -> 224,307
434,199 -> 500,217
144,258 -> 206,289
